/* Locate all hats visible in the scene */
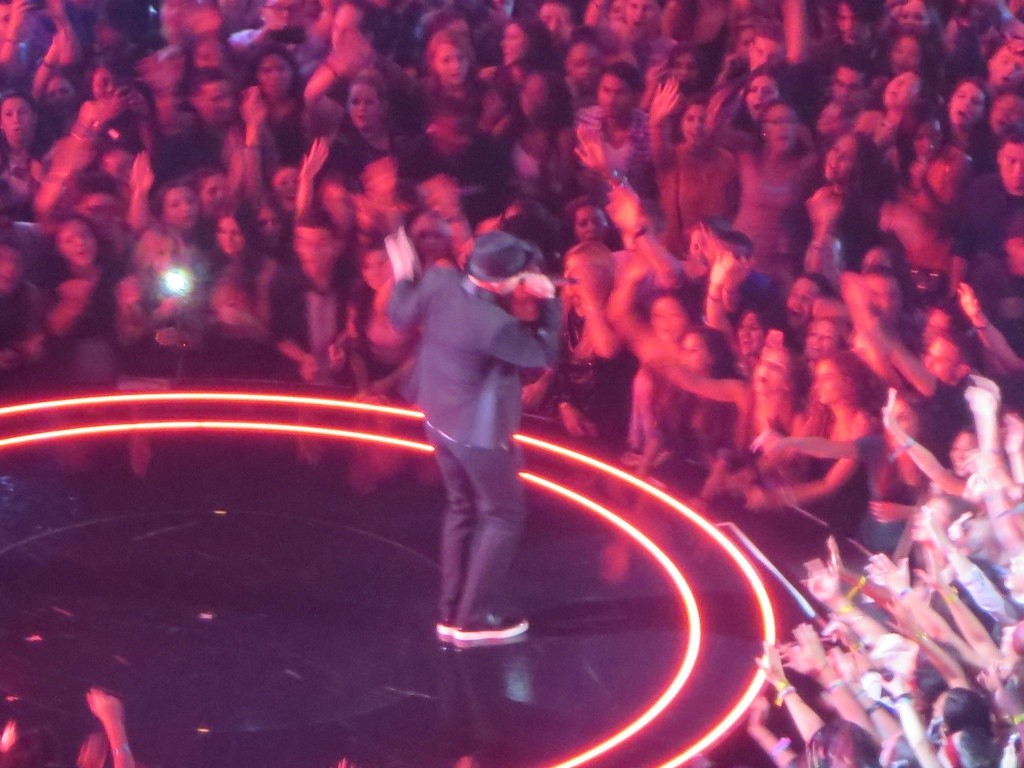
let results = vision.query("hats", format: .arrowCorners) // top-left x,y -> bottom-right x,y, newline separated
464,231 -> 540,280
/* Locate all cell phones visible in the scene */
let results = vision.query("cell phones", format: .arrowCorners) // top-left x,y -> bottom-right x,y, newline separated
114,85 -> 130,97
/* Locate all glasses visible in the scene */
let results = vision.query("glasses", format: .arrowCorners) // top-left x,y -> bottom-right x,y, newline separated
264,4 -> 303,14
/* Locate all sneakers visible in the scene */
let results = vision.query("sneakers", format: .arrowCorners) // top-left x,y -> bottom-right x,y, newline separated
436,613 -> 529,639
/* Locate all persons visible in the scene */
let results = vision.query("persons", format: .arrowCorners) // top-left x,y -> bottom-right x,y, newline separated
383,226 -> 564,641
1,1 -> 1024,768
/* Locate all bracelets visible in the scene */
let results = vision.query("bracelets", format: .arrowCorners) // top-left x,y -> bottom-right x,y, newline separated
839,605 -> 856,612
895,695 -> 915,705
827,681 -> 842,689
107,743 -> 131,754
558,401 -> 571,407
774,680 -> 796,698
903,438 -> 913,449
977,326 -> 996,331
40,59 -> 57,71
325,61 -> 340,82
772,736 -> 792,760
893,587 -> 909,600
811,239 -> 831,250
867,703 -> 882,715
912,632 -> 927,641
707,292 -> 721,301
945,593 -> 958,603
631,226 -> 647,240
5,36 -> 21,44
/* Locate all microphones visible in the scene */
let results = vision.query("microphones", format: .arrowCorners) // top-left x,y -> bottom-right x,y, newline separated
547,277 -> 579,287
